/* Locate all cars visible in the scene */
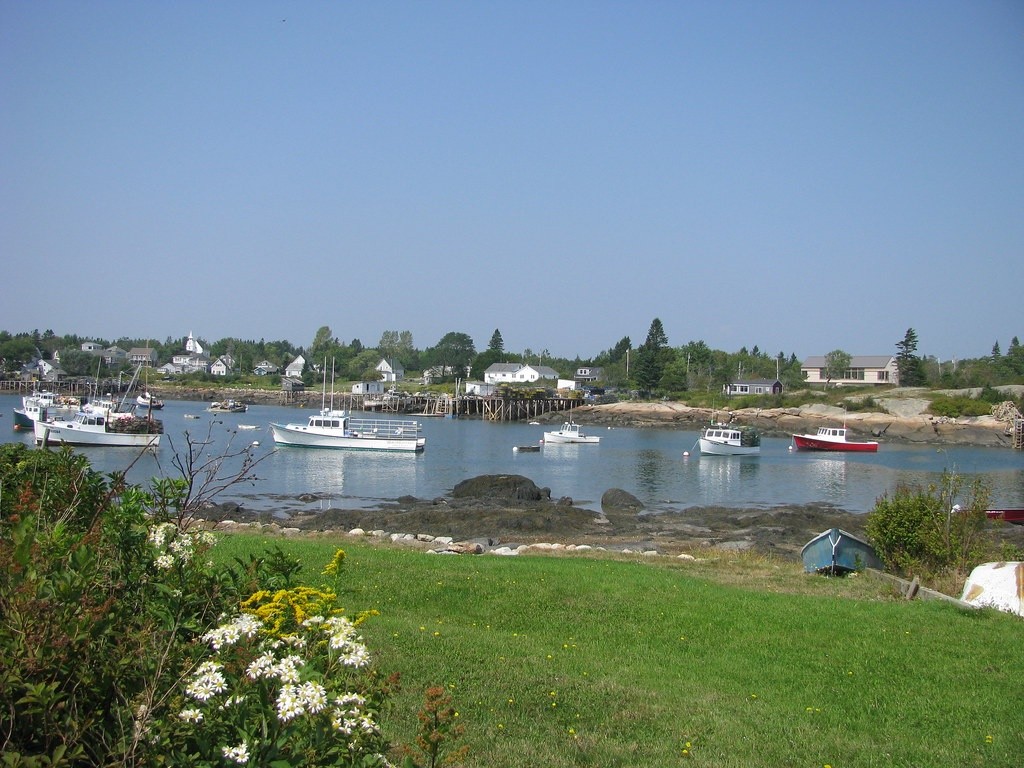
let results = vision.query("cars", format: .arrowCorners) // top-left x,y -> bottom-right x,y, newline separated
576,385 -> 605,396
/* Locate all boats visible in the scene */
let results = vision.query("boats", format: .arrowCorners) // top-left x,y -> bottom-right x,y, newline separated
183,414 -> 200,419
543,412 -> 604,443
513,446 -> 540,453
237,423 -> 260,429
268,356 -> 426,452
697,397 -> 761,456
203,399 -> 249,413
800,528 -> 889,578
792,406 -> 878,453
952,505 -> 1024,526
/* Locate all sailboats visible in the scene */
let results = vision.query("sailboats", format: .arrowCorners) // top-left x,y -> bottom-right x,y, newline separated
12,357 -> 164,447
